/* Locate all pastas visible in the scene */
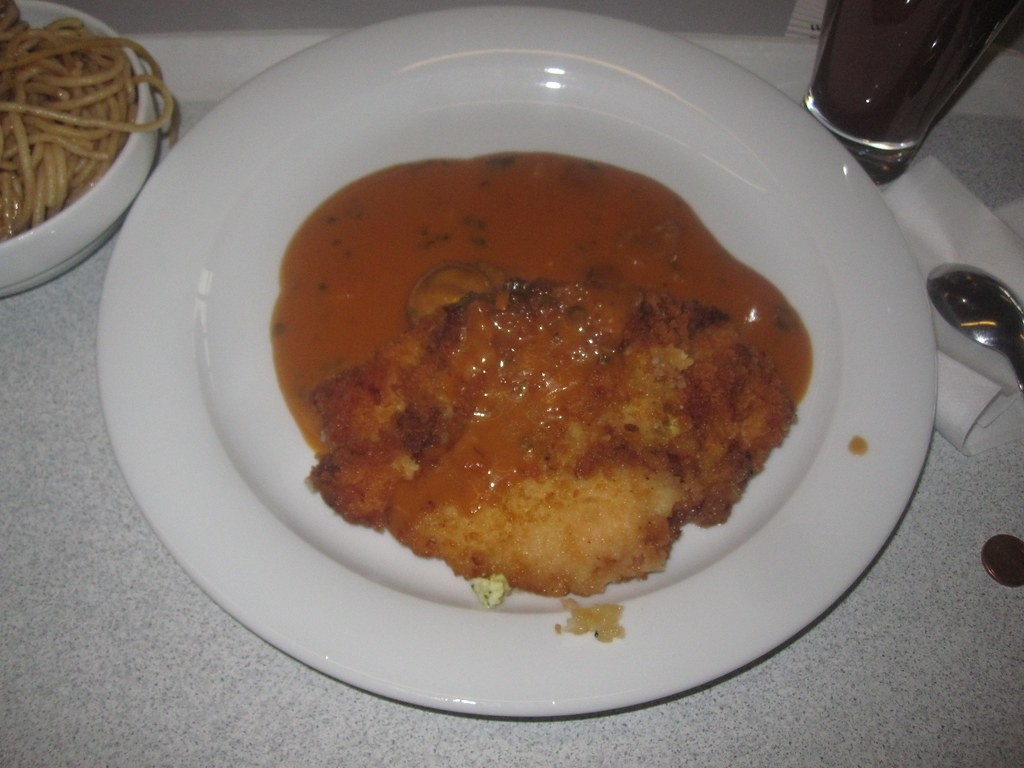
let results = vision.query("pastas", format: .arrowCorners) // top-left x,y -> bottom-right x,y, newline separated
0,0 -> 176,249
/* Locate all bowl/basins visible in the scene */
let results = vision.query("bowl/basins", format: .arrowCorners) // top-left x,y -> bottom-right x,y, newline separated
0,0 -> 158,299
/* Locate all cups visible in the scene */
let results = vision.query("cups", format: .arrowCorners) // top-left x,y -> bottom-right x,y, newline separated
801,0 -> 1024,186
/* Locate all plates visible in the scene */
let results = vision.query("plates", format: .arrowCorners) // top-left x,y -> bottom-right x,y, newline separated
96,5 -> 940,718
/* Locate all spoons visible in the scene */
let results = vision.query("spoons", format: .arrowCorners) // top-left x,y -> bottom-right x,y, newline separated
927,263 -> 1024,394
